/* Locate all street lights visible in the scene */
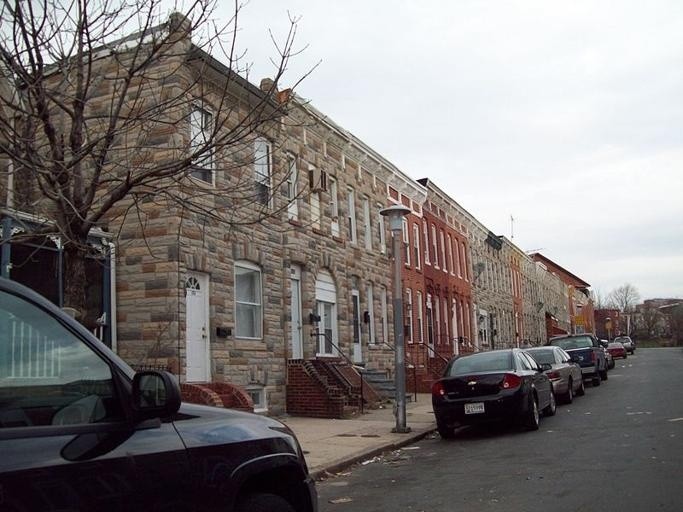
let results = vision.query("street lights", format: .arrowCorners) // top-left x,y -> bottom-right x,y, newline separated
379,202 -> 414,435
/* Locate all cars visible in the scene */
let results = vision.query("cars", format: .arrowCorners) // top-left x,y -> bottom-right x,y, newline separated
601,340 -> 615,369
521,345 -> 585,402
607,342 -> 626,359
613,336 -> 635,355
430,346 -> 555,432
0,269 -> 318,512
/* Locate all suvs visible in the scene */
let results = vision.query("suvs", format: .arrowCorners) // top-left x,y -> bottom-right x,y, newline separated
542,333 -> 607,385
